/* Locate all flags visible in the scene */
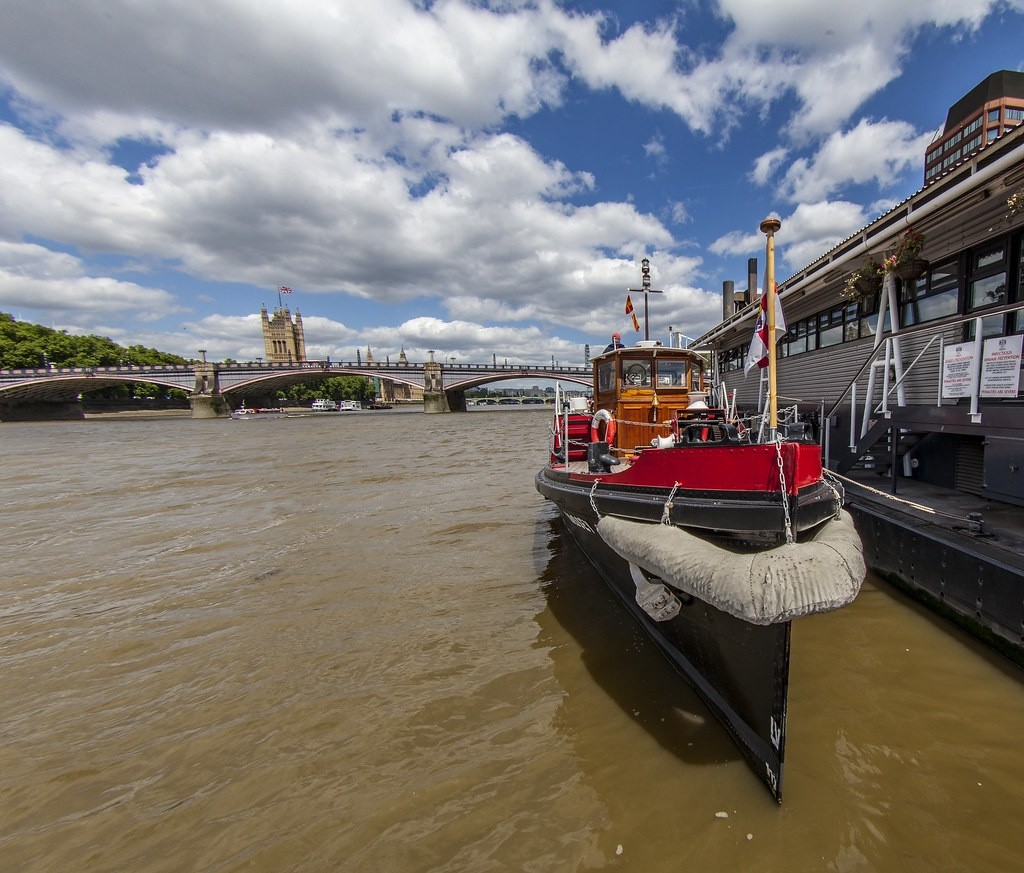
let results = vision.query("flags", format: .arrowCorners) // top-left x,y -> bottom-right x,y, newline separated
278,285 -> 293,295
625,294 -> 640,332
743,244 -> 788,387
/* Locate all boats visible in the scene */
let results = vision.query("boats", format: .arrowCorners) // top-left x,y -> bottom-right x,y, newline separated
312,398 -> 339,412
690,70 -> 1024,654
536,332 -> 865,805
235,400 -> 257,415
367,403 -> 394,409
340,400 -> 362,411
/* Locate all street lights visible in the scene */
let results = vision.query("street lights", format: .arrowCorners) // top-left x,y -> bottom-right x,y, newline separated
627,258 -> 665,341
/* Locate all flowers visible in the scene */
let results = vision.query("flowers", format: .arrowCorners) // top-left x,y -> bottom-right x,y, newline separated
1005,187 -> 1024,223
839,258 -> 892,303
882,230 -> 926,272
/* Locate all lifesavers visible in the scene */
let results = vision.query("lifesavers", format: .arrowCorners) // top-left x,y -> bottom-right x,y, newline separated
590,407 -> 616,447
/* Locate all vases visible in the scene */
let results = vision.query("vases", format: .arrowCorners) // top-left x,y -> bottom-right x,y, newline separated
892,259 -> 930,280
855,278 -> 880,295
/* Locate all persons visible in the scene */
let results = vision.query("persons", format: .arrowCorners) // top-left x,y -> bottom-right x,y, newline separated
598,332 -> 625,391
